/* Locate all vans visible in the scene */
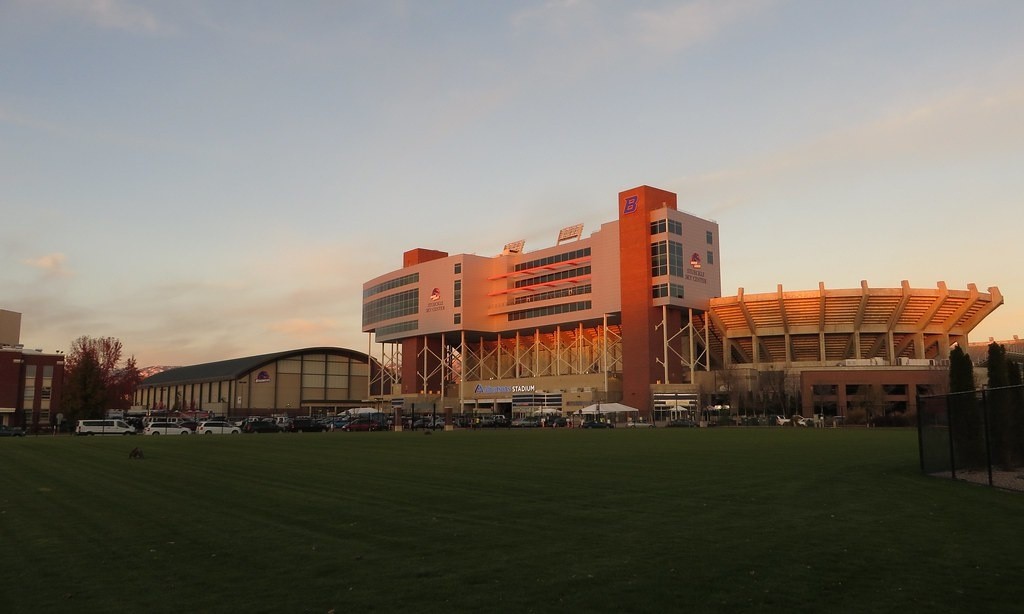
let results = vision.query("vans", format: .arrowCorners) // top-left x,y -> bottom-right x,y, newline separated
77,419 -> 136,437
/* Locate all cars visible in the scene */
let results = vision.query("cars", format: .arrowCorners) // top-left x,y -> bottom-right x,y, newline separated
141,415 -> 700,436
